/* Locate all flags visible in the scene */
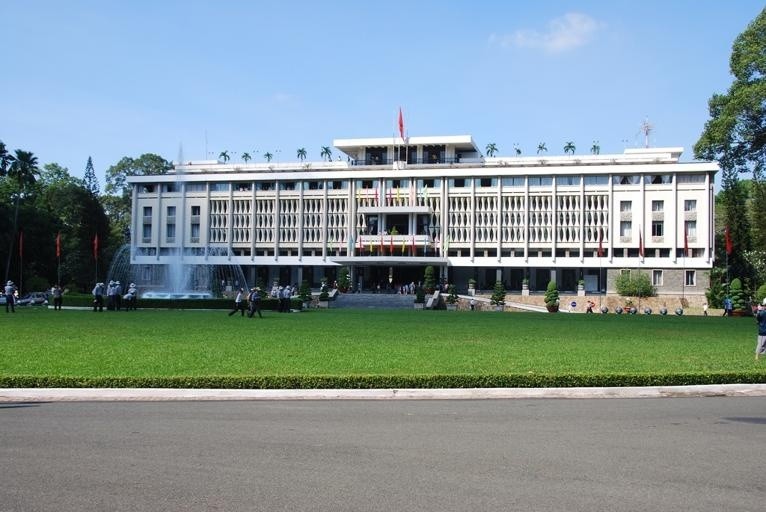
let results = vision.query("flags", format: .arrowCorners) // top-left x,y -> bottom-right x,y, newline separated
330,234 -> 449,253
398,108 -> 405,142
357,184 -> 429,202
93,234 -> 98,258
20,233 -> 23,256
639,228 -> 644,256
726,225 -> 732,255
684,229 -> 688,253
598,236 -> 603,256
56,234 -> 60,257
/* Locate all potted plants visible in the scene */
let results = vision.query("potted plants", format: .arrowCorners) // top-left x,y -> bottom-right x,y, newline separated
543,279 -> 559,312
490,280 -> 507,312
578,280 -> 585,290
522,279 -> 530,290
468,278 -> 477,289
289,279 -> 313,313
445,283 -> 460,311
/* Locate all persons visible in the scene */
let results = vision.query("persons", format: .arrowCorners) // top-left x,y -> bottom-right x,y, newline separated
54,285 -> 62,310
471,299 -> 474,310
5,281 -> 18,312
723,302 -> 732,317
436,281 -> 449,293
91,281 -> 136,311
586,301 -> 592,313
229,282 -> 299,318
703,303 -> 707,316
399,282 -> 417,296
752,298 -> 766,359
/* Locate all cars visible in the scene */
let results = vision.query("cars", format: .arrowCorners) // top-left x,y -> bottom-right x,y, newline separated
0,292 -> 48,305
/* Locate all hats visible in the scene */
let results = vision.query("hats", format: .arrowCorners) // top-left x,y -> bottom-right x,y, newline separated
97,280 -> 135,288
6,280 -> 14,286
239,287 -> 262,291
272,285 -> 291,290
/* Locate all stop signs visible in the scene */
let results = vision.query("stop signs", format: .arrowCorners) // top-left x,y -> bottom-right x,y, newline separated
591,303 -> 595,307
